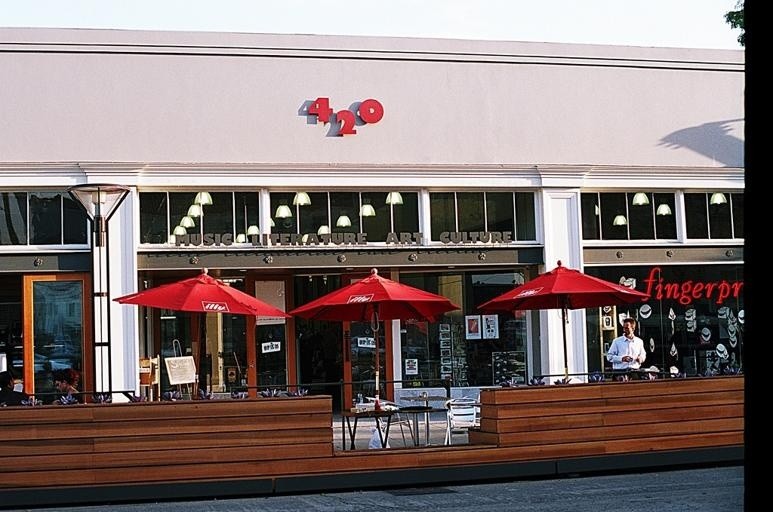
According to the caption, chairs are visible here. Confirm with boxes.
[442,397,482,446]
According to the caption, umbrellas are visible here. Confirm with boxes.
[285,267,463,403]
[475,259,653,384]
[109,267,293,394]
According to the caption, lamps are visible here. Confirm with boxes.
[168,192,403,243]
[612,192,727,227]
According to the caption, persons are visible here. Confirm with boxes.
[606,316,647,381]
[4,331,18,378]
[0,369,32,409]
[50,368,82,406]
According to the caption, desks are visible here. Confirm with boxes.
[341,396,454,450]
